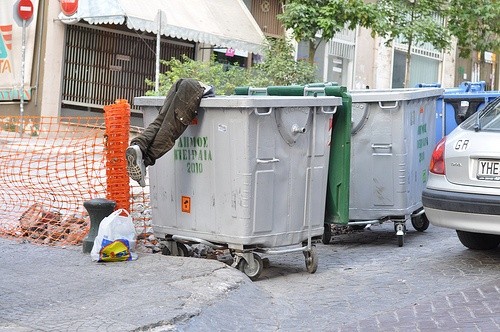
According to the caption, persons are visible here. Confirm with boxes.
[125,79,215,187]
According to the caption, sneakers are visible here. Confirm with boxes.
[124,143,146,186]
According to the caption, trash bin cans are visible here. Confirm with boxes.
[346,87,444,249]
[416,80,500,145]
[133,82,352,281]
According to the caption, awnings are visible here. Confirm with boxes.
[57,0,271,58]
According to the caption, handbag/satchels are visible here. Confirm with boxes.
[90,209,139,262]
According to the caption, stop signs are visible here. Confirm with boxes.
[17,0,33,20]
[60,0,78,16]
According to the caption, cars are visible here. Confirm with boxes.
[422,95,500,253]
